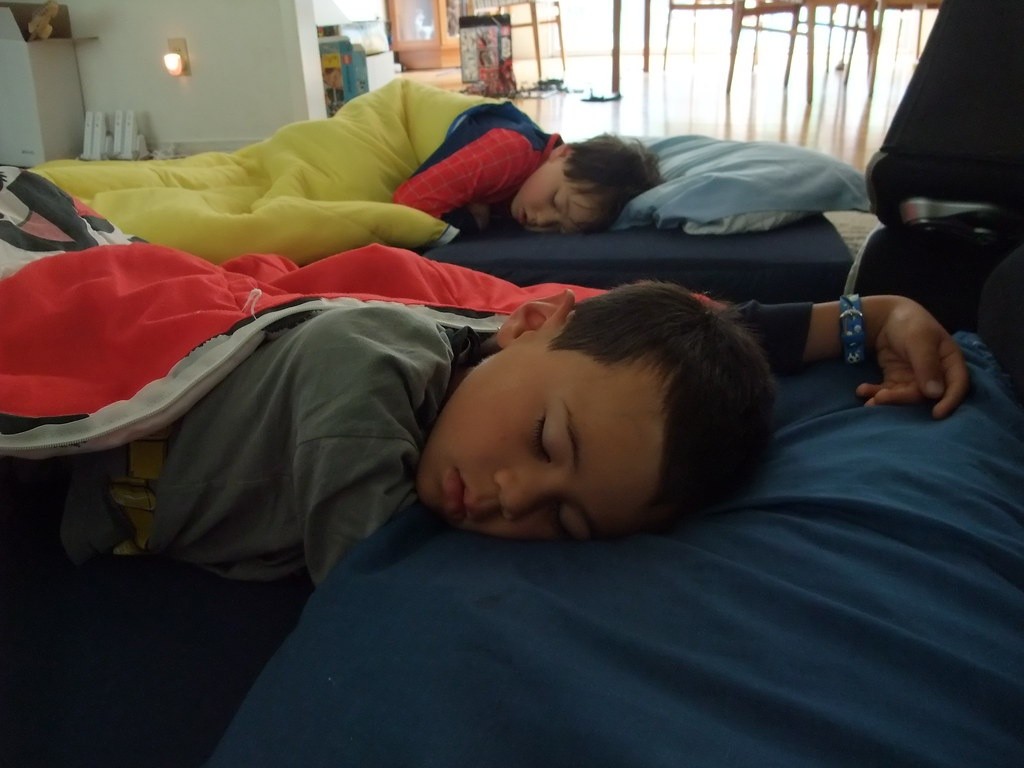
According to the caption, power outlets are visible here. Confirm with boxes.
[168,38,191,76]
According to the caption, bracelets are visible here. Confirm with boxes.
[840,290,868,364]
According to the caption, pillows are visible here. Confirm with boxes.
[610,135,871,235]
[224,330,1024,768]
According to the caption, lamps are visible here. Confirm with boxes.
[313,0,352,37]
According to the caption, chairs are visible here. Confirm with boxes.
[661,0,943,107]
[463,0,566,77]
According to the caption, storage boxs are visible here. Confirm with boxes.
[318,36,369,118]
[0,0,99,166]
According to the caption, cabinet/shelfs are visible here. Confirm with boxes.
[388,0,473,70]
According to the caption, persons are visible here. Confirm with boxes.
[1,279,968,591]
[393,102,663,234]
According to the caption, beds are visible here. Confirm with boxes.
[0,210,854,302]
[0,329,1023,767]
